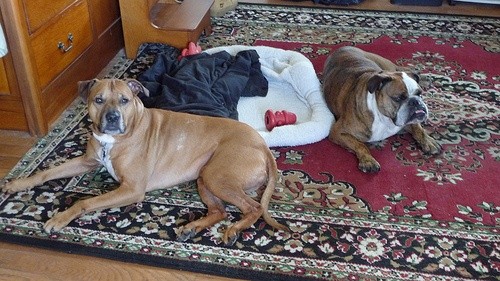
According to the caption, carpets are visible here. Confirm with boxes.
[0,4,500,281]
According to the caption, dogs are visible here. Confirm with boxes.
[0,78,294,246]
[321,46,444,174]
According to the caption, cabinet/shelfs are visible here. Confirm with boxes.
[0,0,215,138]
